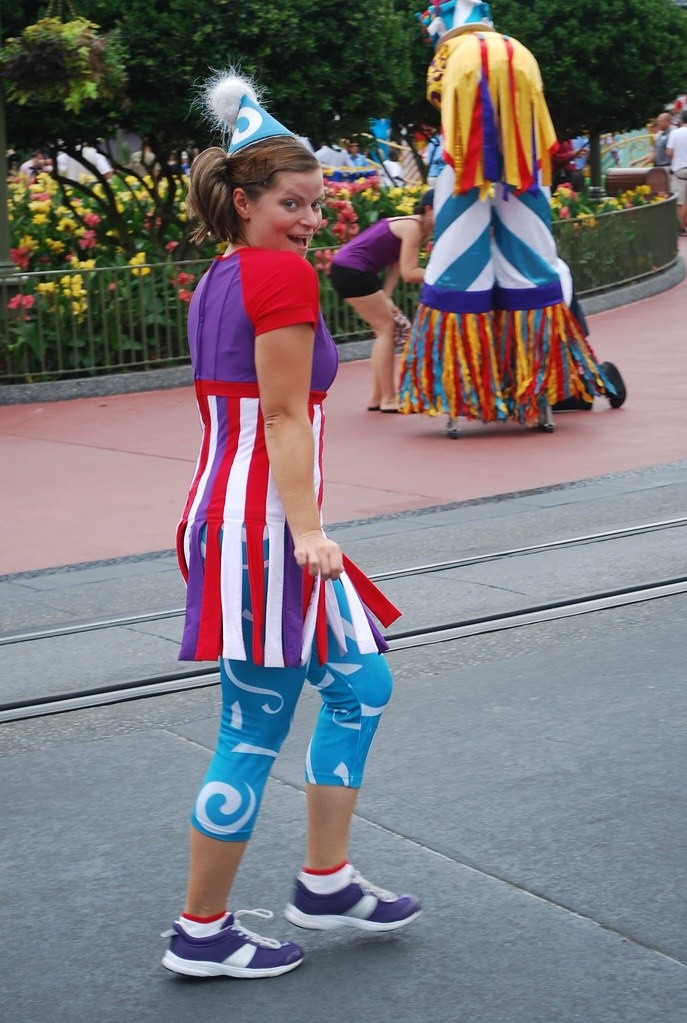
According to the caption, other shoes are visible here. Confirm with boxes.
[600,360,627,408]
[551,396,592,412]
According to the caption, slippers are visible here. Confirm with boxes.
[382,407,398,413]
[368,405,379,411]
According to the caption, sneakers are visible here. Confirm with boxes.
[283,871,424,932]
[160,909,305,978]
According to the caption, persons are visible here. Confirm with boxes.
[6,136,198,187]
[313,121,445,187]
[329,188,434,413]
[159,135,425,978]
[647,94,687,237]
[548,134,591,192]
